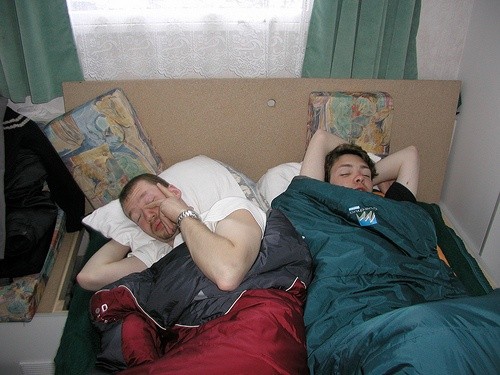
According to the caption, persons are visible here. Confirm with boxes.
[77,173,307,375]
[300,129,480,375]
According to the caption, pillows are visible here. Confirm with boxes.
[255,153,383,207]
[45,87,168,210]
[303,91,393,163]
[82,154,272,251]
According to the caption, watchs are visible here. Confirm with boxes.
[177,210,200,226]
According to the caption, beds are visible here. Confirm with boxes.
[54,80,500,375]
